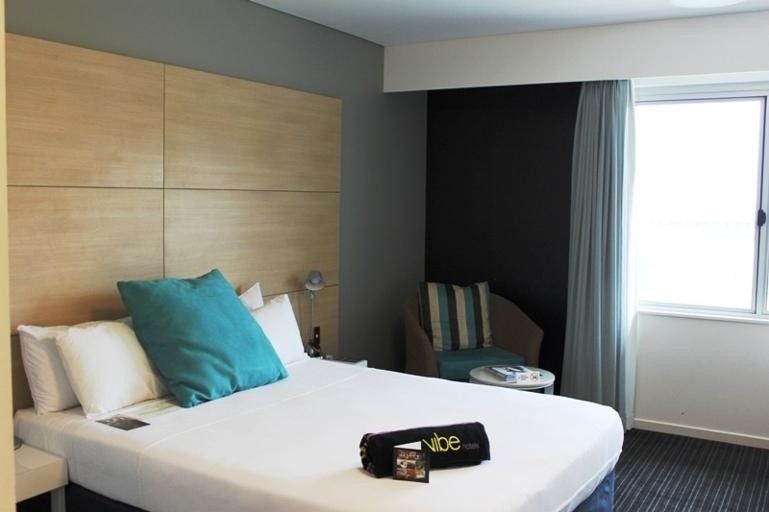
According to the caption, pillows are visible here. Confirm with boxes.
[251,293,306,365]
[238,281,263,309]
[417,280,493,352]
[116,267,288,408]
[17,316,136,417]
[54,320,173,419]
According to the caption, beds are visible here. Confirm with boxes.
[16,358,624,511]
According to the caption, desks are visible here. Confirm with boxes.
[14,443,68,512]
[469,365,555,394]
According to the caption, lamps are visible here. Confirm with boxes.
[302,268,327,358]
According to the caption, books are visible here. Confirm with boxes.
[393,440,430,484]
[485,363,545,382]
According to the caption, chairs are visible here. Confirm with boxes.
[401,287,544,384]
